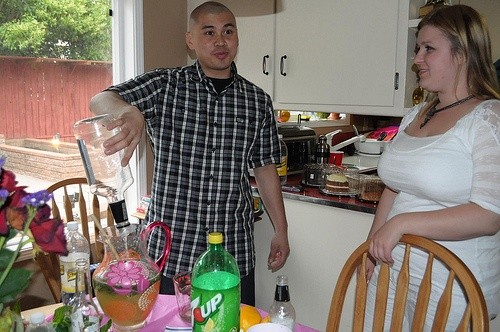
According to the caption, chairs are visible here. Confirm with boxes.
[325,234,489,332]
[35,178,119,304]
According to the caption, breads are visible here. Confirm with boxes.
[360,179,385,201]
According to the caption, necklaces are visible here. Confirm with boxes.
[419,93,475,130]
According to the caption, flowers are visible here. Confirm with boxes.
[0,154,69,332]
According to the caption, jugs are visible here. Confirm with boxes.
[92,221,171,330]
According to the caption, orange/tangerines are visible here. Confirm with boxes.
[240,304,262,332]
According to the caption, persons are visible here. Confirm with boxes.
[357,4,500,331]
[88,1,290,306]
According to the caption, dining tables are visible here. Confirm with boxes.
[20,294,320,332]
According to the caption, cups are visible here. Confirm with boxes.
[172,273,192,319]
[247,323,293,332]
[329,151,344,166]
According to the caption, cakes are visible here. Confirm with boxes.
[326,174,349,193]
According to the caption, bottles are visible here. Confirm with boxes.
[191,233,241,332]
[67,258,101,332]
[268,275,296,332]
[412,71,424,107]
[25,312,56,332]
[316,135,329,164]
[58,221,90,308]
[75,115,134,234]
[275,134,288,185]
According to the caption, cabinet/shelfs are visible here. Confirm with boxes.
[404,0,451,116]
[186,0,399,117]
